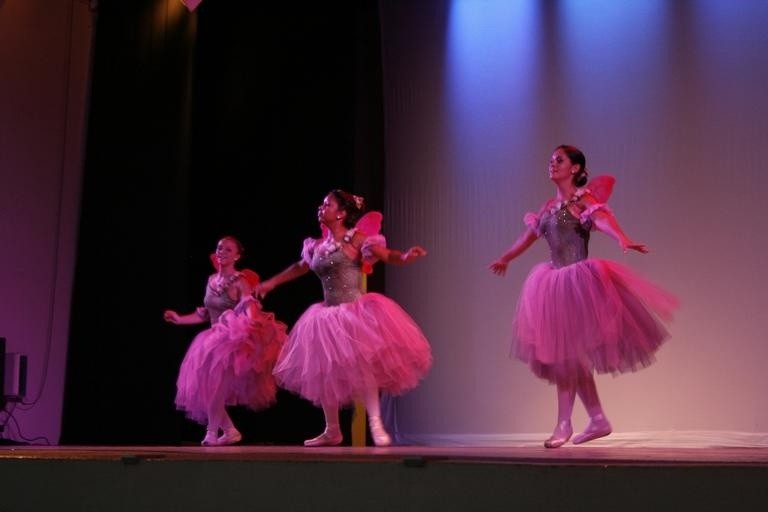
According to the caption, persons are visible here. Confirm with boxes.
[251,187,435,448]
[486,142,680,450]
[163,235,291,446]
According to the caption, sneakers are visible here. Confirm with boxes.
[200,424,220,447]
[543,419,572,448]
[217,425,242,446]
[368,416,392,447]
[572,413,612,445]
[303,423,343,448]
